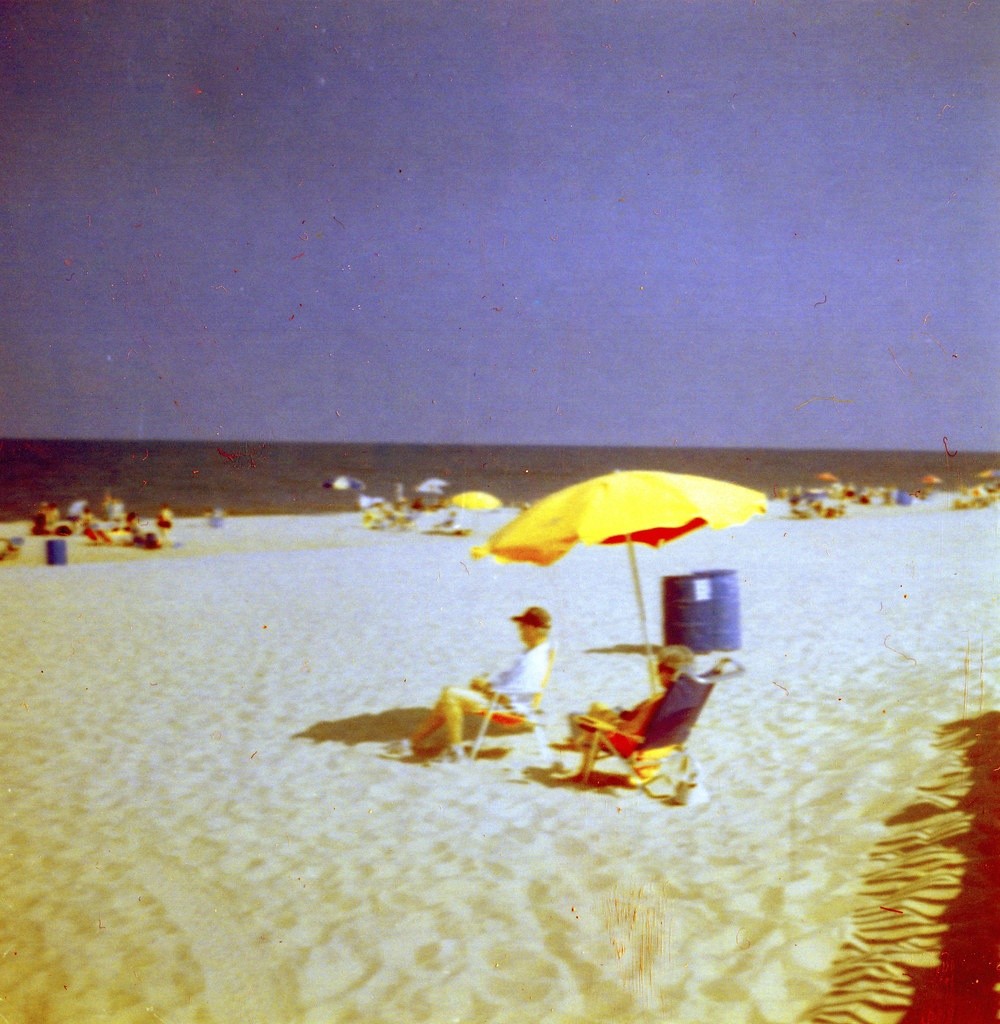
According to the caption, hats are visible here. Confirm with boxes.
[511,607,552,627]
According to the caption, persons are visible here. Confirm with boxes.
[415,607,552,767]
[30,494,174,545]
[363,496,465,532]
[569,645,695,755]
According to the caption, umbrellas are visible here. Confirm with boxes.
[974,469,1000,479]
[322,475,366,492]
[469,468,768,695]
[922,475,940,484]
[816,472,837,488]
[452,491,502,512]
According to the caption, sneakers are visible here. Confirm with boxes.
[428,746,468,767]
[381,740,415,758]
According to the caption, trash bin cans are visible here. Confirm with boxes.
[47,539,66,566]
[896,490,911,506]
[692,568,745,654]
[660,573,718,656]
[210,506,224,527]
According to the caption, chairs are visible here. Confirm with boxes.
[573,668,717,798]
[467,650,554,759]
